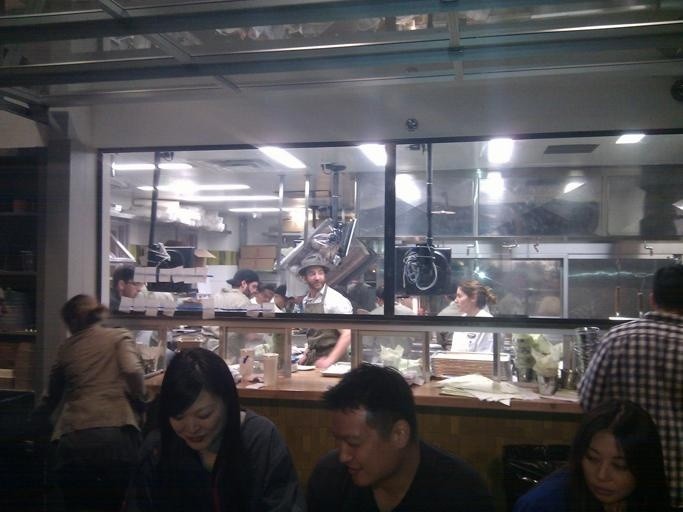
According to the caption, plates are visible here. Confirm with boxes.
[0,340,32,391]
[0,288,35,333]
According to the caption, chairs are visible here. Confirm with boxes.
[501,440,571,509]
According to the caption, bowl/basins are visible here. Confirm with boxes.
[513,326,600,397]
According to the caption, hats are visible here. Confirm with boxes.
[298,255,329,274]
[227,269,257,285]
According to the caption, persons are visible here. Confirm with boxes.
[29,294,153,512]
[512,399,670,511]
[139,346,298,511]
[305,362,490,512]
[494,268,636,319]
[578,266,682,512]
[112,255,493,372]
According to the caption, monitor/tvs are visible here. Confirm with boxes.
[395,247,451,295]
[147,248,194,292]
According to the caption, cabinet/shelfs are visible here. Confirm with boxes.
[0,148,48,392]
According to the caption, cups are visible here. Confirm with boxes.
[238,347,278,388]
